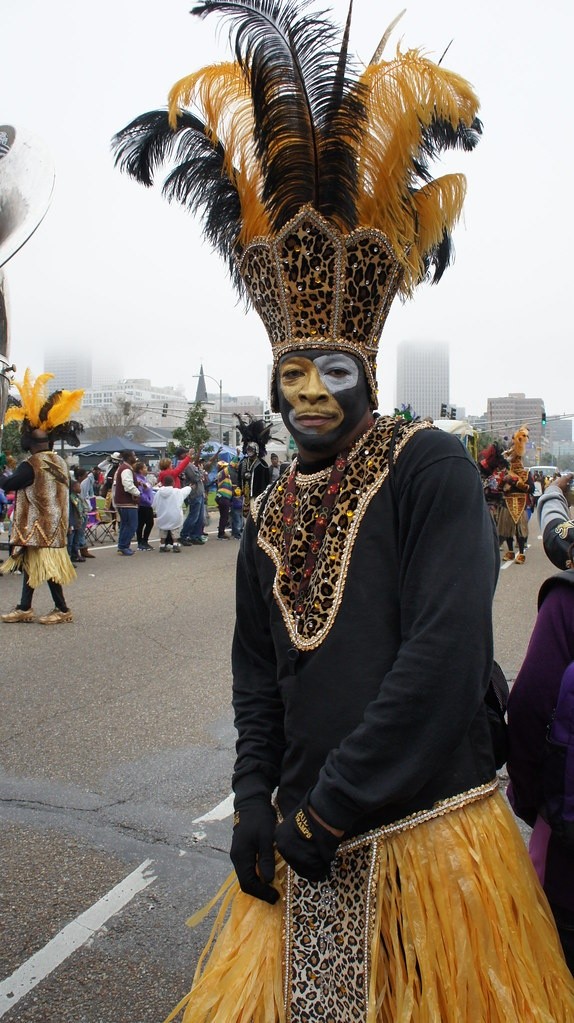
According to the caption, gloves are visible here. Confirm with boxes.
[276,786,341,881]
[230,807,280,904]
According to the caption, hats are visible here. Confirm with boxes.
[173,447,189,455]
[217,460,228,470]
[195,458,206,466]
[110,452,124,460]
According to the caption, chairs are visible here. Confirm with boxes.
[82,511,118,547]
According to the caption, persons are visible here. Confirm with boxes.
[477,455,561,564]
[537,473,574,571]
[68,442,297,565]
[505,564,574,979]
[0,428,77,624]
[180,349,574,1023]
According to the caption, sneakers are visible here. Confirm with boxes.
[1,606,37,622]
[39,607,72,625]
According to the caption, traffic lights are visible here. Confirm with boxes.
[450,407,456,420]
[541,413,546,425]
[440,403,447,417]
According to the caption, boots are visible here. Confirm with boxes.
[80,543,96,558]
[72,545,86,562]
[72,563,78,568]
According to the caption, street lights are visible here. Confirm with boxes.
[192,375,222,446]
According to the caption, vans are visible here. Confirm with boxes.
[530,466,558,476]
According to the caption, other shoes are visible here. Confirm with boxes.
[158,527,244,552]
[117,548,136,556]
[516,554,525,563]
[503,551,515,560]
[523,542,527,548]
[515,541,520,548]
[144,541,155,549]
[137,544,151,551]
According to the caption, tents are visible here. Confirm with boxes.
[71,436,159,456]
[200,442,249,492]
